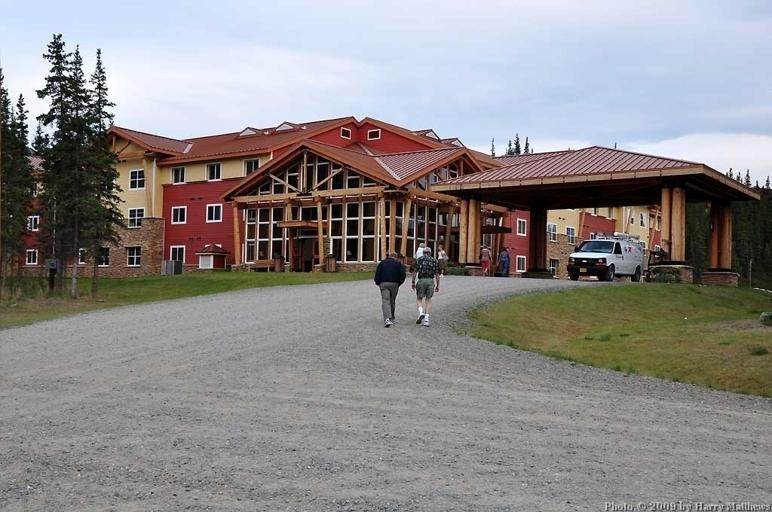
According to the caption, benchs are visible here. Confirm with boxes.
[247,260,275,272]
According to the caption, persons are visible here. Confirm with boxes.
[374,251,406,327]
[481,246,493,276]
[438,244,446,277]
[411,247,440,326]
[499,249,509,277]
[416,243,425,258]
[506,247,511,277]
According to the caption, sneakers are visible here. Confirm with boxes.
[420,319,429,326]
[383,317,396,327]
[416,312,425,323]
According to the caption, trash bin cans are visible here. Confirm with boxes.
[274,255,285,272]
[326,254,336,272]
[161,260,182,275]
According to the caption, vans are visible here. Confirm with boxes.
[566,238,644,281]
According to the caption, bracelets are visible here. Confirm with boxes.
[412,280,415,284]
